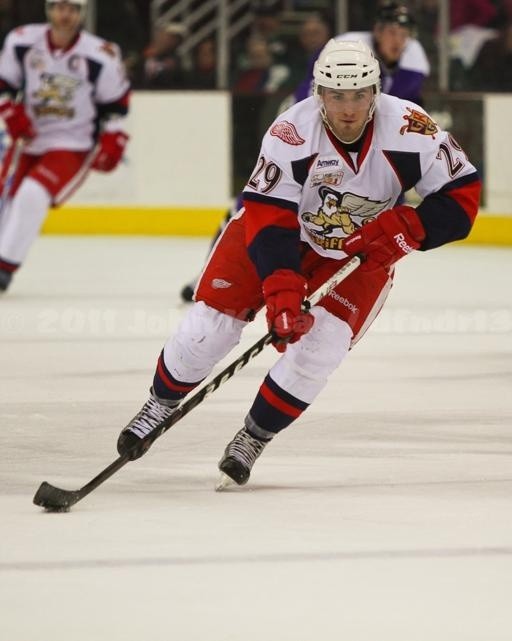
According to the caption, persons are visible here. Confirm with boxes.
[3,2,135,301]
[181,0,434,303]
[119,1,430,175]
[112,36,483,484]
[448,0,512,93]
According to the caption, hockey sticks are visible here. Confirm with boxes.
[34,252,364,512]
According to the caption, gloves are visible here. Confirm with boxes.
[0,95,39,141]
[89,120,132,173]
[259,269,316,353]
[338,206,428,274]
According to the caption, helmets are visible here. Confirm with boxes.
[375,4,419,37]
[311,39,382,124]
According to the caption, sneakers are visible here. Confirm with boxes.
[117,386,180,463]
[216,425,274,486]
